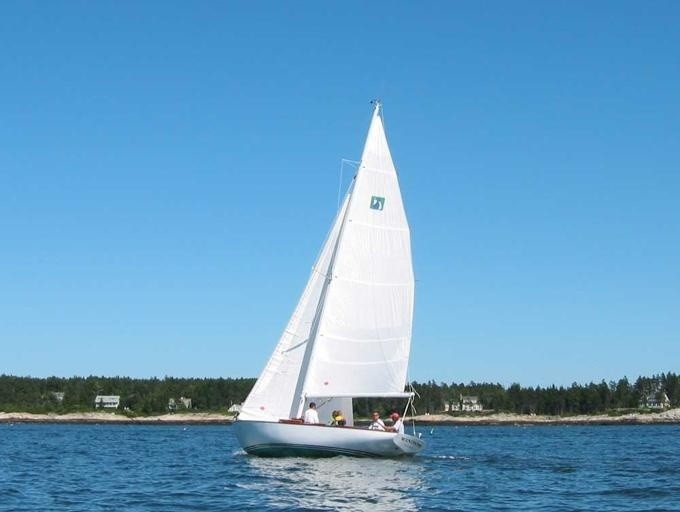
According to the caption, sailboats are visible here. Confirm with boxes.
[230,95,429,458]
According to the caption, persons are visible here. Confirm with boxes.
[327,410,346,426]
[304,402,319,424]
[368,410,385,431]
[382,413,404,434]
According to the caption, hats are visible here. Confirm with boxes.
[390,413,399,418]
